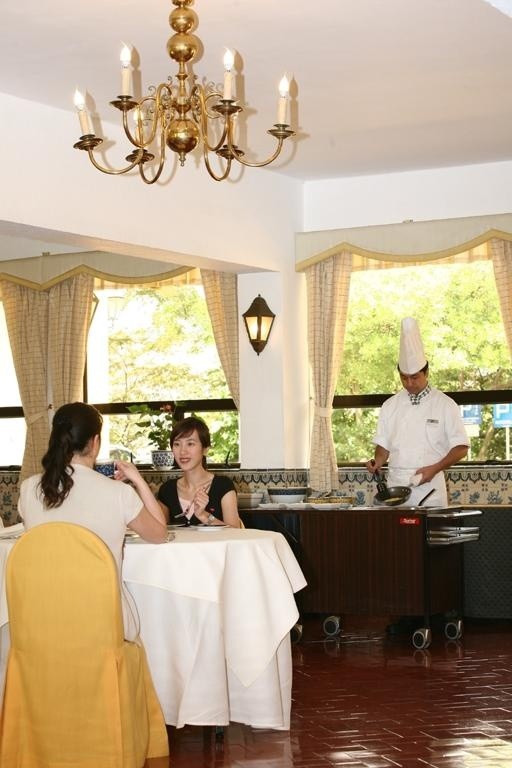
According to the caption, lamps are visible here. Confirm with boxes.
[70,0,297,183]
[243,292,276,356]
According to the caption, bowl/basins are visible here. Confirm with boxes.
[308,497,330,503]
[330,496,355,504]
[267,487,306,504]
[236,494,264,509]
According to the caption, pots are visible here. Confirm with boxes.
[372,483,414,506]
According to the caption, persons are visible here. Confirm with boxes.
[16,401,168,644]
[365,318,469,510]
[155,415,241,528]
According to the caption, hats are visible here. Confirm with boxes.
[398,316,428,375]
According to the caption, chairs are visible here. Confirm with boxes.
[0,521,169,767]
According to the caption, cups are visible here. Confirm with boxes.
[95,462,115,478]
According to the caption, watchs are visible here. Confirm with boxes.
[204,513,216,526]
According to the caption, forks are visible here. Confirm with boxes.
[174,488,203,519]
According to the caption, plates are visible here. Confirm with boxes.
[285,503,312,509]
[259,503,285,509]
[313,504,342,510]
[125,530,140,538]
[191,523,230,531]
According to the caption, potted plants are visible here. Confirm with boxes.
[126,397,189,470]
